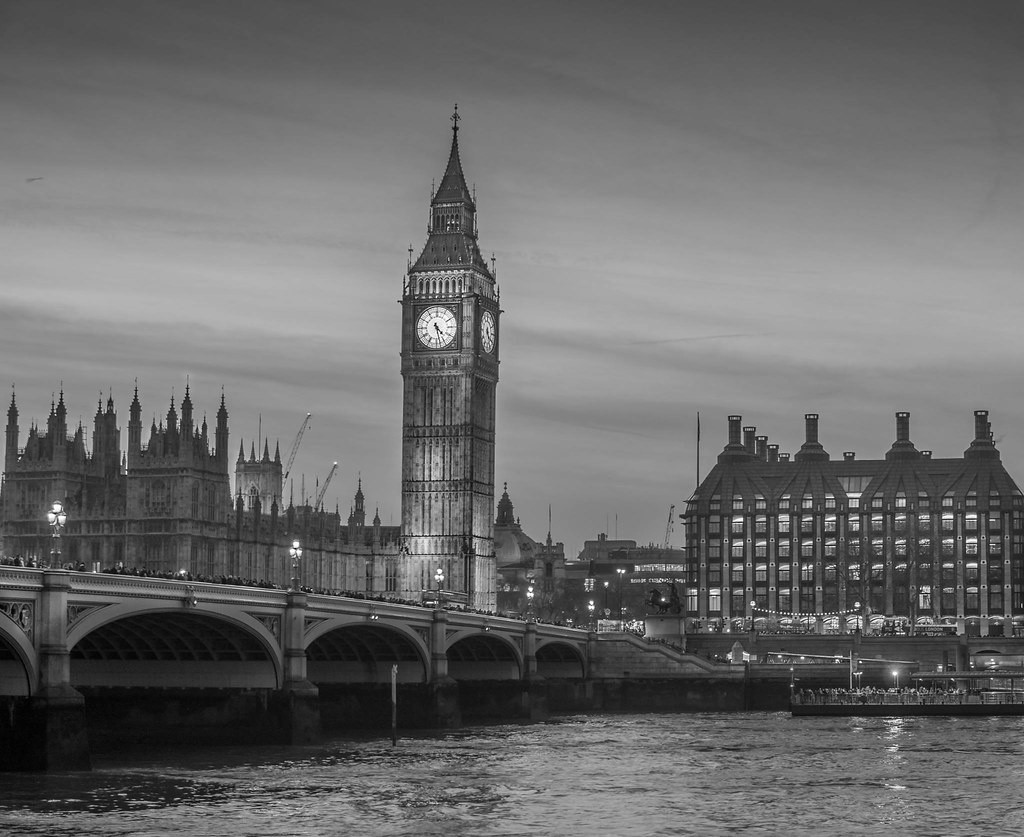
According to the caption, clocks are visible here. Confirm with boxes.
[481,310,496,354]
[415,305,457,350]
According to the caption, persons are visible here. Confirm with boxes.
[891,620,899,636]
[300,585,597,632]
[799,686,970,704]
[0,554,276,590]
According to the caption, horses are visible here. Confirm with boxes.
[644,588,672,615]
